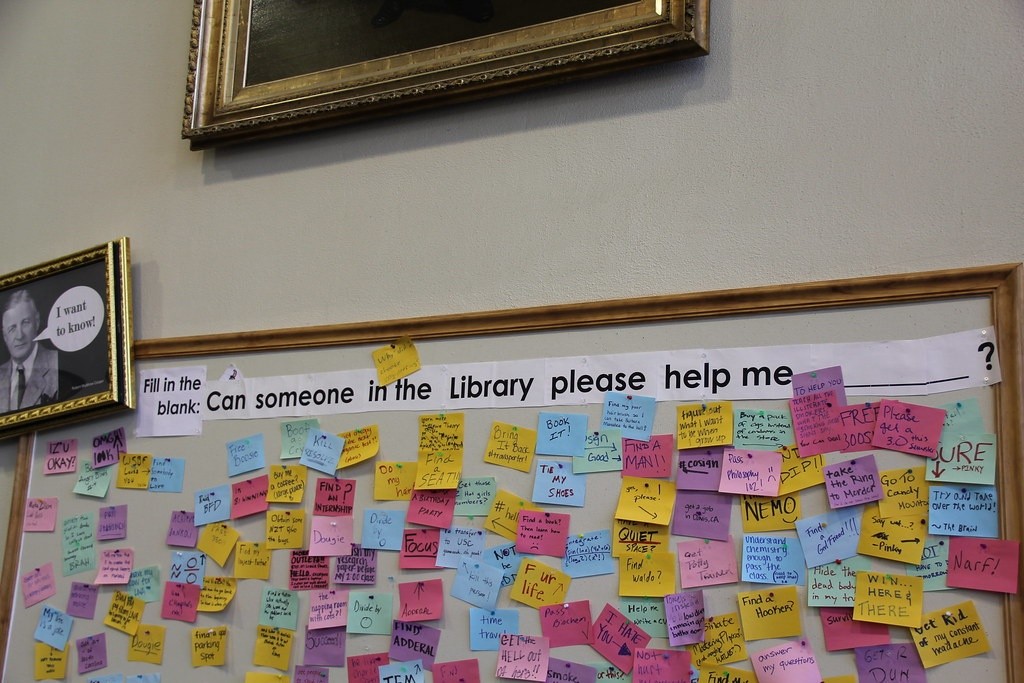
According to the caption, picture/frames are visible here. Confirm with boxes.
[0,236,133,437]
[1,263,1022,683]
[180,0,708,149]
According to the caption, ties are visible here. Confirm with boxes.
[16,365,25,410]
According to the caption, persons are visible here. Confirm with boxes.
[0,289,59,414]
